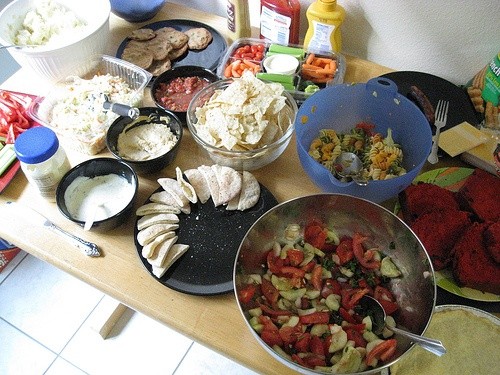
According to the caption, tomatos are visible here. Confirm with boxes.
[241,224,402,373]
[233,43,265,62]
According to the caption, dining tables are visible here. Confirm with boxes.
[1,1,500,375]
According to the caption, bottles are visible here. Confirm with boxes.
[259,0,300,47]
[303,0,348,57]
[225,0,251,43]
[15,126,73,202]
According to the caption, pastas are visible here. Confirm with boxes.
[306,126,407,182]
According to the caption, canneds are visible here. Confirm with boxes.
[225,0,251,41]
[302,0,347,53]
[257,0,300,47]
[15,126,71,203]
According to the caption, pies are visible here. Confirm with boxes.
[136,164,260,279]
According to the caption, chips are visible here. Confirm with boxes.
[192,72,294,151]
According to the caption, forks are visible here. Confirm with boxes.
[425,99,449,163]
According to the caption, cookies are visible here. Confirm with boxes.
[121,26,213,76]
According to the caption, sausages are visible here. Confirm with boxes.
[411,85,435,121]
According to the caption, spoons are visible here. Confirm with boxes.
[353,290,447,357]
[333,150,369,186]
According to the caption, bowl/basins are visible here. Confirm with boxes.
[186,76,299,170]
[106,104,184,176]
[293,76,434,206]
[55,156,139,234]
[150,65,222,126]
[110,0,169,22]
[230,187,438,373]
[0,0,112,81]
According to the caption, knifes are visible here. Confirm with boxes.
[6,201,104,259]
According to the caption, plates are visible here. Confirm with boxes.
[394,163,500,307]
[118,18,229,86]
[377,301,500,374]
[370,72,478,163]
[136,167,281,294]
[217,34,348,104]
